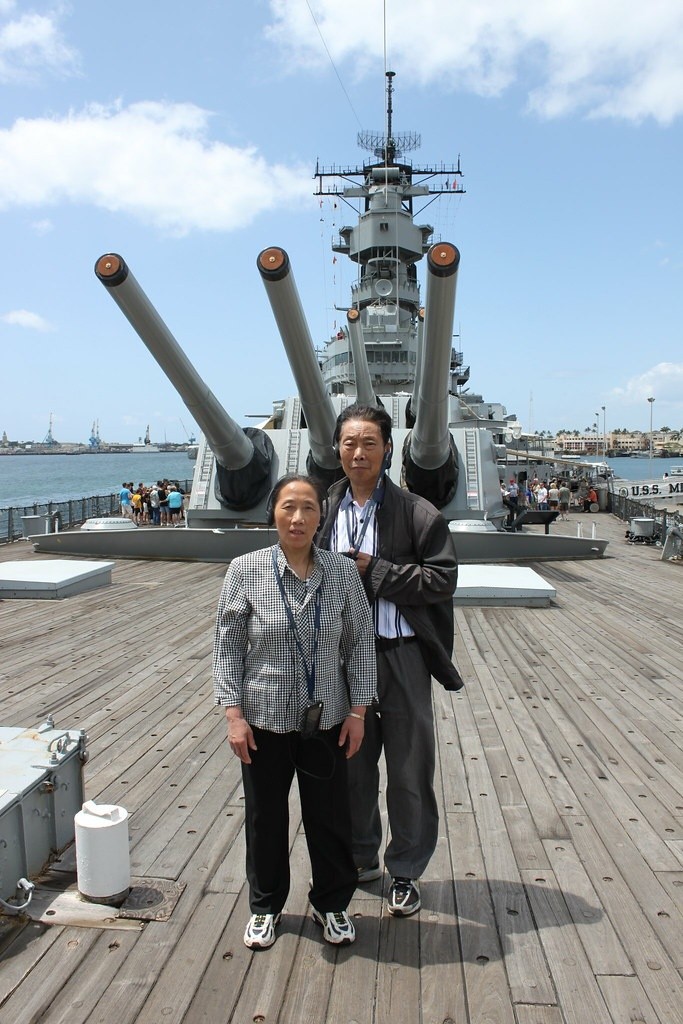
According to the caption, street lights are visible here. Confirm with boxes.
[594,412,599,462]
[601,408,606,460]
[646,397,654,459]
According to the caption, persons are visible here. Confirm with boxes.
[548,483,559,522]
[508,479,519,512]
[118,479,185,528]
[559,483,571,522]
[526,486,534,513]
[661,472,674,499]
[500,483,510,508]
[212,474,379,950]
[313,402,465,918]
[535,482,548,511]
[583,485,597,513]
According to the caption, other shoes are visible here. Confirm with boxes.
[134,520,182,528]
[181,517,186,521]
[584,509,590,514]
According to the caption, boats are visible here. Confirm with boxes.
[561,454,580,459]
[670,465,683,477]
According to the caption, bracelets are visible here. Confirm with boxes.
[347,712,364,721]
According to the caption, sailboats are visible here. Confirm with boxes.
[127,424,161,453]
[175,417,201,452]
[36,411,67,454]
[78,419,111,453]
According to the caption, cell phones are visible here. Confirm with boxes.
[303,702,325,740]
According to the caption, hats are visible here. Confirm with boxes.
[500,482,506,488]
[152,486,157,490]
[509,479,515,484]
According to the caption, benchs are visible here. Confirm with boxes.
[590,503,599,512]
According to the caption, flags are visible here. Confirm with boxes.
[446,179,449,190]
[452,178,456,190]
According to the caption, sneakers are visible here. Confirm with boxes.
[308,905,357,945]
[387,877,422,918]
[308,858,382,890]
[243,909,284,950]
[560,517,570,521]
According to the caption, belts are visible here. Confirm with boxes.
[373,636,422,651]
